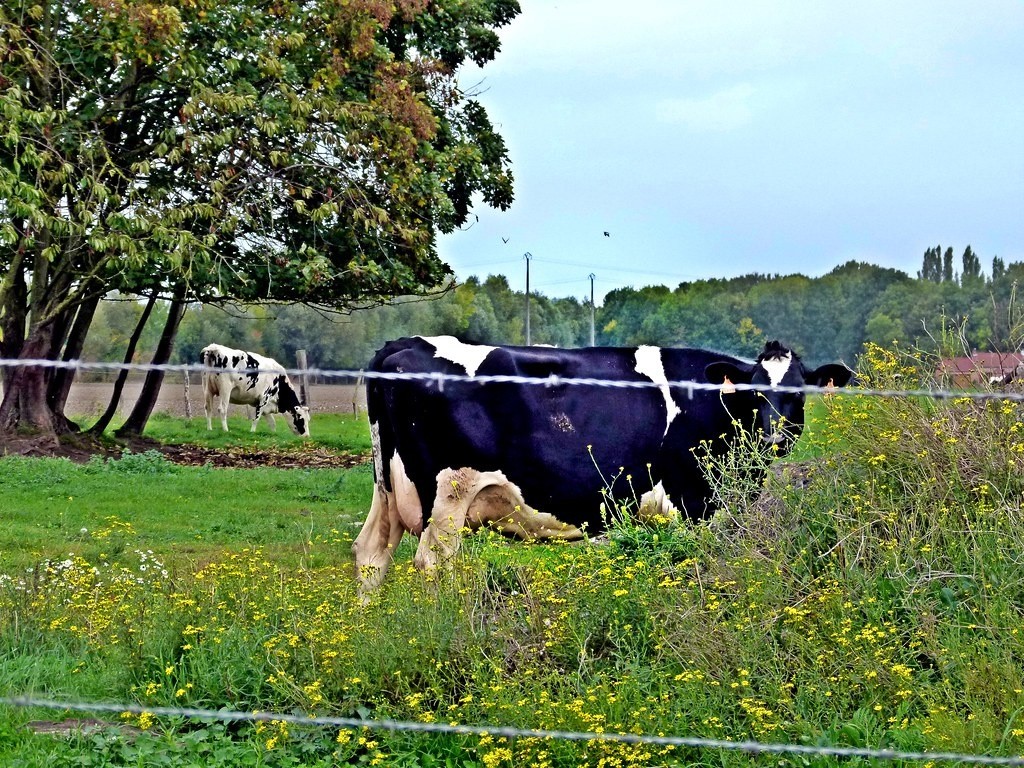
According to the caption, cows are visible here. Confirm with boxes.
[197,335,849,610]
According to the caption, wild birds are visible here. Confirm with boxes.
[603,231,610,237]
